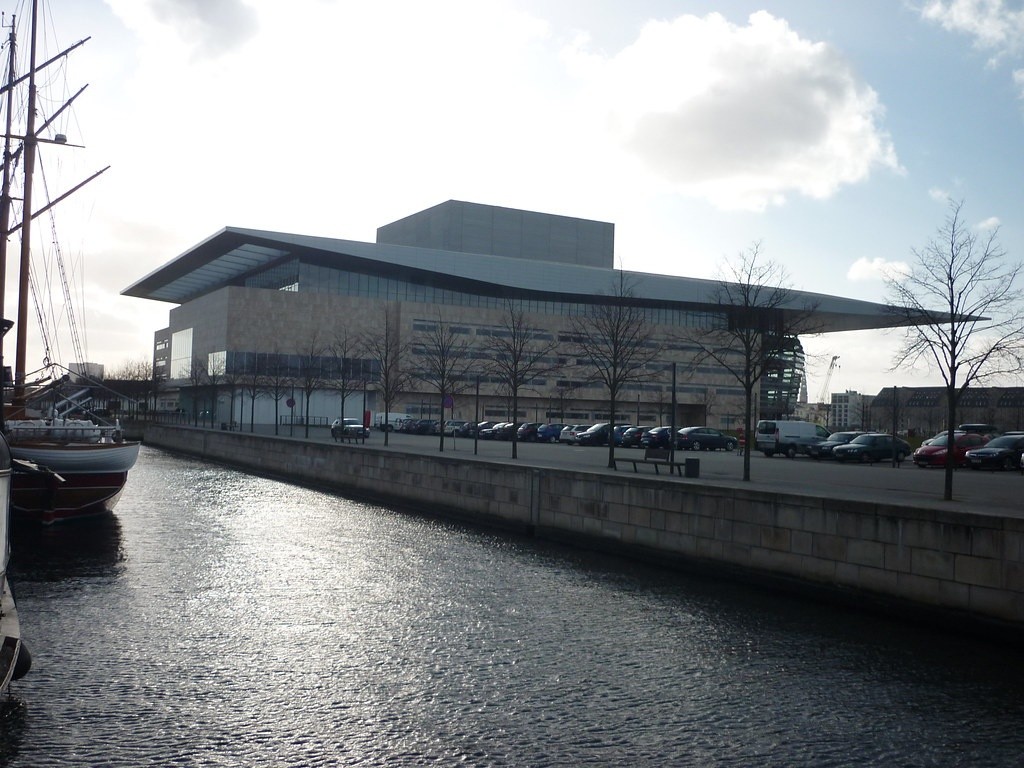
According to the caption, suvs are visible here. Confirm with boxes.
[515,422,543,441]
[559,425,592,443]
[621,426,652,449]
[537,423,567,443]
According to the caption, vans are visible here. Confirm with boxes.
[753,418,832,458]
[575,423,632,446]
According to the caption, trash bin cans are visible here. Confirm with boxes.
[220,423,227,430]
[685,458,700,478]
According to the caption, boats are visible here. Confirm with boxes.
[7,409,142,521]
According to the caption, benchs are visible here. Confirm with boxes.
[611,445,685,476]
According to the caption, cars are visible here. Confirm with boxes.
[431,421,446,434]
[674,426,737,451]
[470,421,498,437]
[607,425,631,443]
[914,424,1024,473]
[413,420,437,433]
[480,422,509,438]
[493,423,517,438]
[815,431,911,463]
[459,422,477,436]
[332,418,371,439]
[639,425,684,447]
[401,418,417,432]
[444,420,465,434]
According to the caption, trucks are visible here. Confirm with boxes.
[376,413,414,431]
